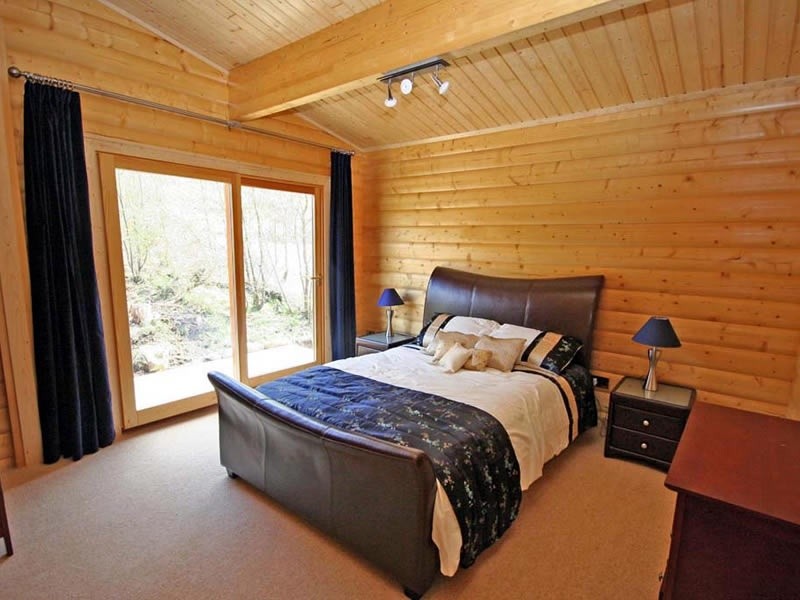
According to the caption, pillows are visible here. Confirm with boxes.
[416,312,585,371]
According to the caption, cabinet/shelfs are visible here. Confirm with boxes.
[656,400,800,600]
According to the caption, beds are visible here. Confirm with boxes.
[208,265,607,600]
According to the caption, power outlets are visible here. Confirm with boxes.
[592,374,610,390]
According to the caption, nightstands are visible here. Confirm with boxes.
[355,332,411,356]
[603,375,697,474]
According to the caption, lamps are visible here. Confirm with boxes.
[383,79,397,108]
[399,72,419,95]
[375,288,405,337]
[632,314,682,391]
[429,64,451,97]
